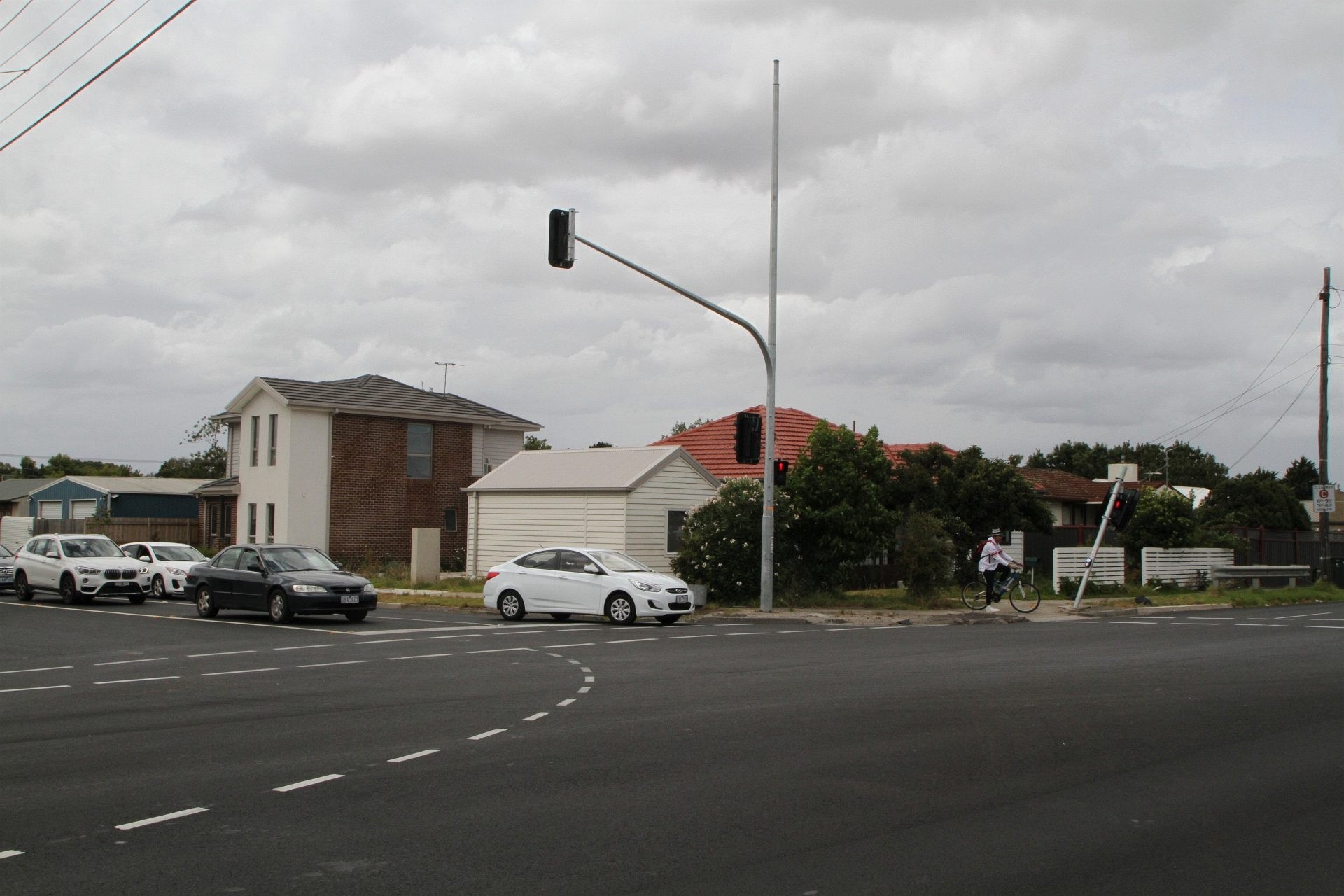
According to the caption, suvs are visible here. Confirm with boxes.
[13,533,153,607]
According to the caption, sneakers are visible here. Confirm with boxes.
[1003,586,1010,591]
[985,606,1000,612]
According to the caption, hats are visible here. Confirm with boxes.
[991,529,1003,536]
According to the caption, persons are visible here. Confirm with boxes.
[978,528,1022,612]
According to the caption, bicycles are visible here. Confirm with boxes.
[962,565,1041,614]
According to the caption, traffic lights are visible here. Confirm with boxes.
[776,459,789,486]
[732,412,762,466]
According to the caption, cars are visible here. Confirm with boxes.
[115,541,213,599]
[183,543,378,623]
[0,542,17,591]
[483,546,696,626]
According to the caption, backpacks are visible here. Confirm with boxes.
[971,539,994,563]
